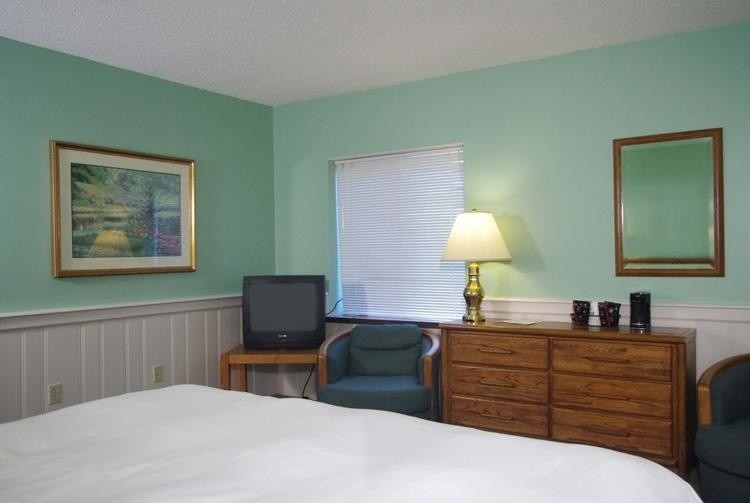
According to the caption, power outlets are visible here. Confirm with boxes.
[47,381,63,405]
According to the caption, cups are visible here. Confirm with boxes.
[629,291,650,330]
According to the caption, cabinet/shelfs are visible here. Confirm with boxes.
[219,345,318,391]
[440,318,697,480]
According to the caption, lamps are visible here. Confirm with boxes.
[439,208,514,323]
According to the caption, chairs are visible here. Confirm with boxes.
[687,349,750,503]
[319,322,442,423]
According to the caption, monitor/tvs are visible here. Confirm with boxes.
[242,275,325,350]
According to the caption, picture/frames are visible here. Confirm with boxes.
[50,136,198,279]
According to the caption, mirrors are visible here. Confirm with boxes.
[613,127,726,278]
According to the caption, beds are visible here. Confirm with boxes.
[0,382,708,503]
